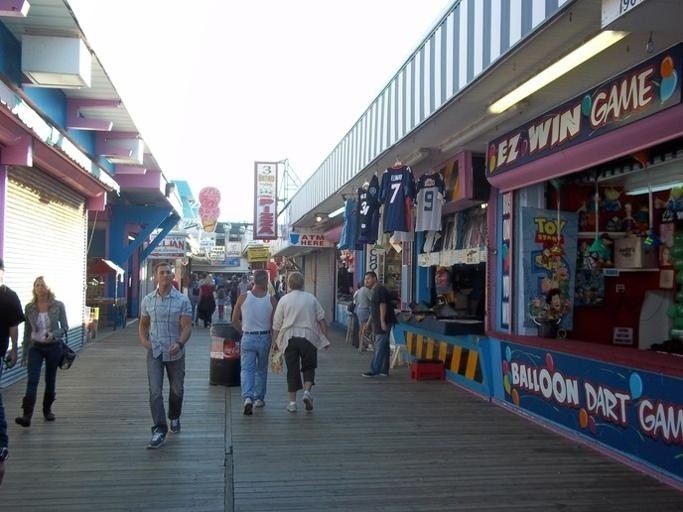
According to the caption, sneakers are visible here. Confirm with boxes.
[303,394,313,411]
[169,419,180,434]
[287,403,297,411]
[361,371,389,377]
[148,432,167,448]
[243,398,264,414]
[0,447,10,460]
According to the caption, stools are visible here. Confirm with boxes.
[411,359,445,380]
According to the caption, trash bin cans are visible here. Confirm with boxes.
[209,321,243,388]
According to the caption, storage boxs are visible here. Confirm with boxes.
[615,237,658,269]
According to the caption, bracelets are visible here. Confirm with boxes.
[175,339,185,349]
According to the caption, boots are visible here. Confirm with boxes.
[42,394,57,420]
[15,398,35,428]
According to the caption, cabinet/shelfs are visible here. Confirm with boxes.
[577,232,660,271]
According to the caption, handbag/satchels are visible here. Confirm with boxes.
[60,344,77,369]
[347,303,356,312]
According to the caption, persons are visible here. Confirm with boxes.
[230,268,276,416]
[13,275,68,425]
[357,270,397,380]
[270,272,328,415]
[0,259,26,463]
[181,271,376,352]
[136,260,194,451]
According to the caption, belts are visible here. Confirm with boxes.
[246,330,270,335]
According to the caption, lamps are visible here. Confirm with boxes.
[315,213,328,221]
[646,30,655,53]
[489,29,631,114]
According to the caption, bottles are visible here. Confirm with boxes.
[2,363,12,370]
[2,351,10,363]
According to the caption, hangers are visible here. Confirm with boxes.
[394,155,403,167]
[362,175,369,188]
